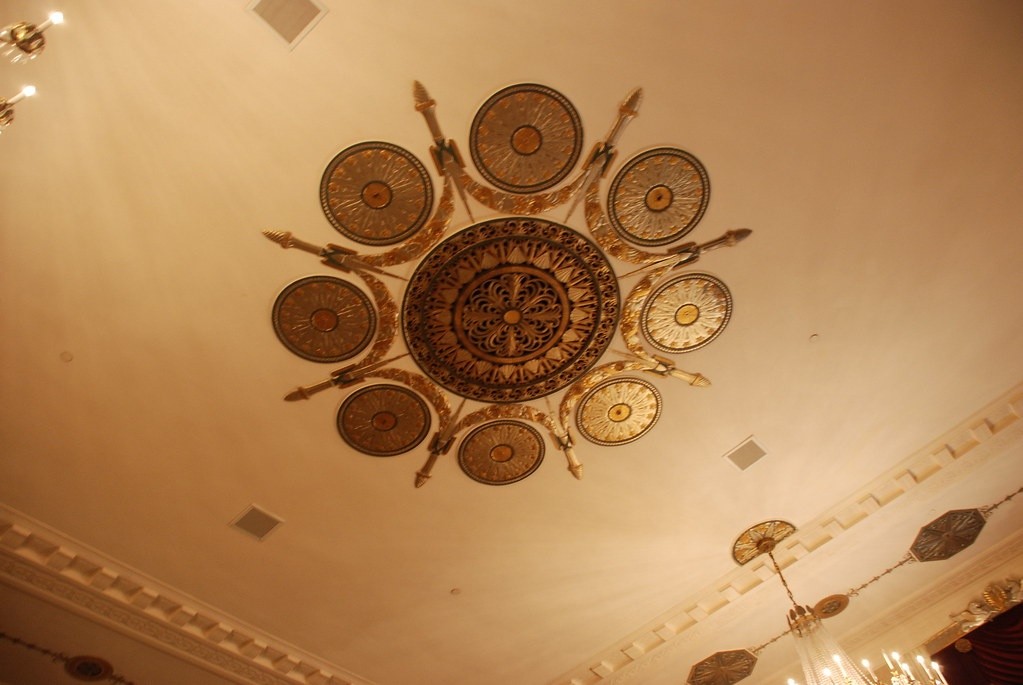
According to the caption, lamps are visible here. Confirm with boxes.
[0,9,68,128]
[733,521,948,685]
